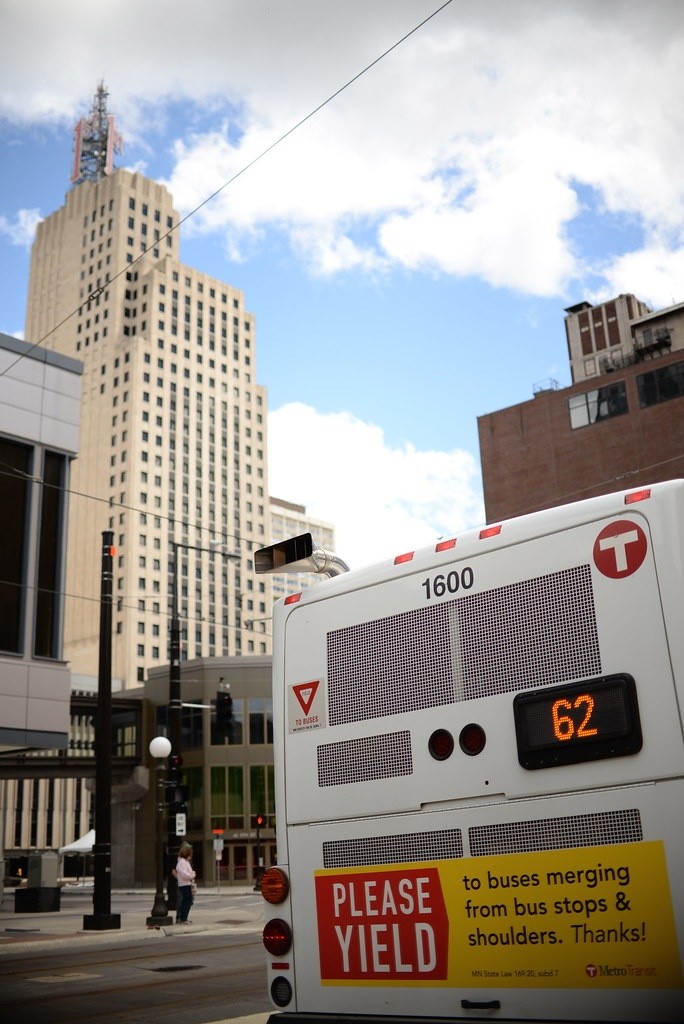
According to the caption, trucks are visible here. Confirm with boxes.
[250,479,682,1024]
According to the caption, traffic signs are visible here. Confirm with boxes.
[173,812,187,837]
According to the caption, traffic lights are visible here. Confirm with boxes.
[168,755,184,783]
[167,785,189,807]
[215,690,235,739]
[256,814,263,829]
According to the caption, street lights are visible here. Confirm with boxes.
[145,735,173,928]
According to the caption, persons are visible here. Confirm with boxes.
[171,841,197,925]
